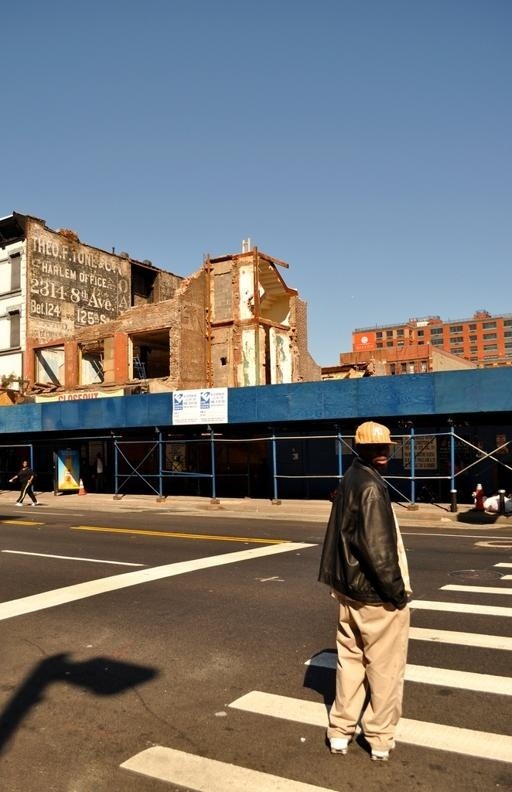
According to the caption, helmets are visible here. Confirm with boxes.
[353,419,396,446]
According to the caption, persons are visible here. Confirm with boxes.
[92,451,106,493]
[9,459,39,506]
[318,422,413,764]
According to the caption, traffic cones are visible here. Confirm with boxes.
[469,482,487,513]
[77,476,87,496]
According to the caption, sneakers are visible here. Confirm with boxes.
[31,501,39,508]
[370,739,396,762]
[327,737,350,756]
[15,501,25,509]
